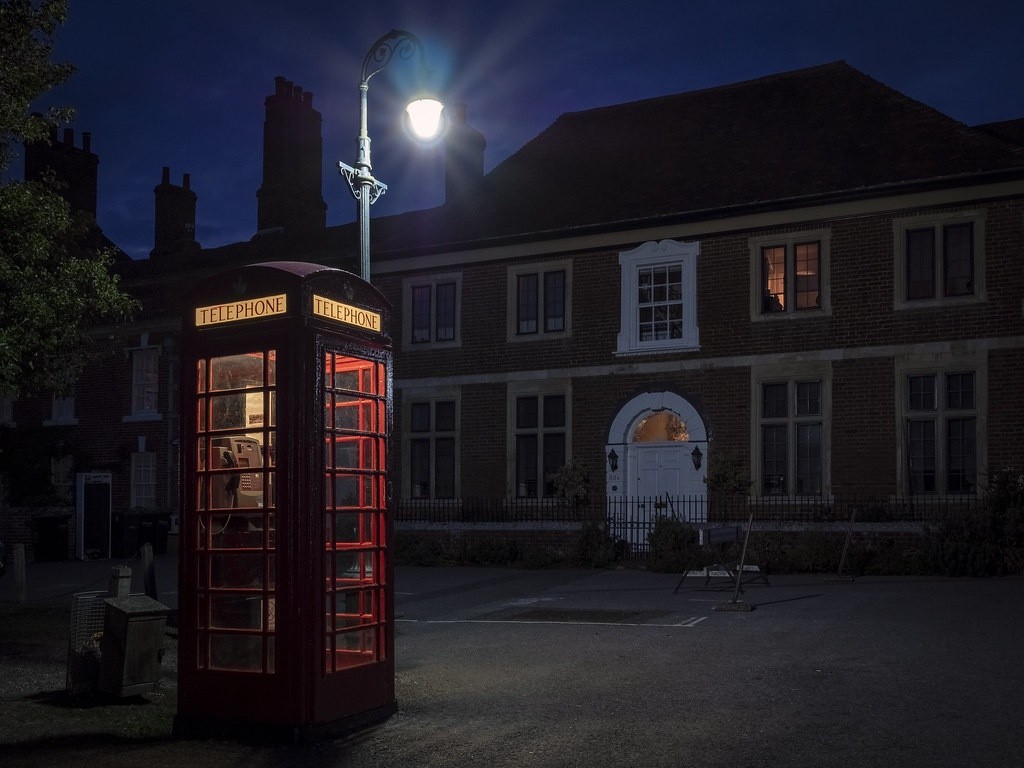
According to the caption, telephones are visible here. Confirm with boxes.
[209,435,273,518]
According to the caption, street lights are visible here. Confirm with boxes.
[335,28,459,281]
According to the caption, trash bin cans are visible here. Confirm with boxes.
[66,591,110,702]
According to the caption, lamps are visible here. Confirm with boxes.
[690,444,703,471]
[607,447,619,472]
